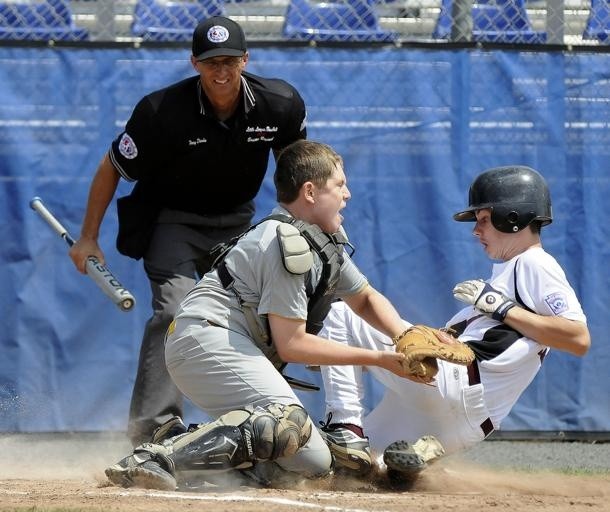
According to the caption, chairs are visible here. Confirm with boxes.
[0,0,90,41]
[433,0,547,43]
[282,0,400,42]
[130,0,224,42]
[583,0,610,47]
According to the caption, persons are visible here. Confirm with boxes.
[69,16,307,452]
[105,139,476,491]
[309,165,592,489]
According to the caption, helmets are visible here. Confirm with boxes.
[451,163,555,234]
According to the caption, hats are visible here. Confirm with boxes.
[191,14,248,63]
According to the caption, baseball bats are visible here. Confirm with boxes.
[29,197,136,312]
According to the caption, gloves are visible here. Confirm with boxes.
[451,277,520,323]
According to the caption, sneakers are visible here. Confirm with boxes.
[378,432,446,492]
[147,414,190,445]
[104,449,179,491]
[313,425,373,479]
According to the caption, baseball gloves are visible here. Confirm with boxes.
[376,324,476,386]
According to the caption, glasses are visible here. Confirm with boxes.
[200,58,244,69]
